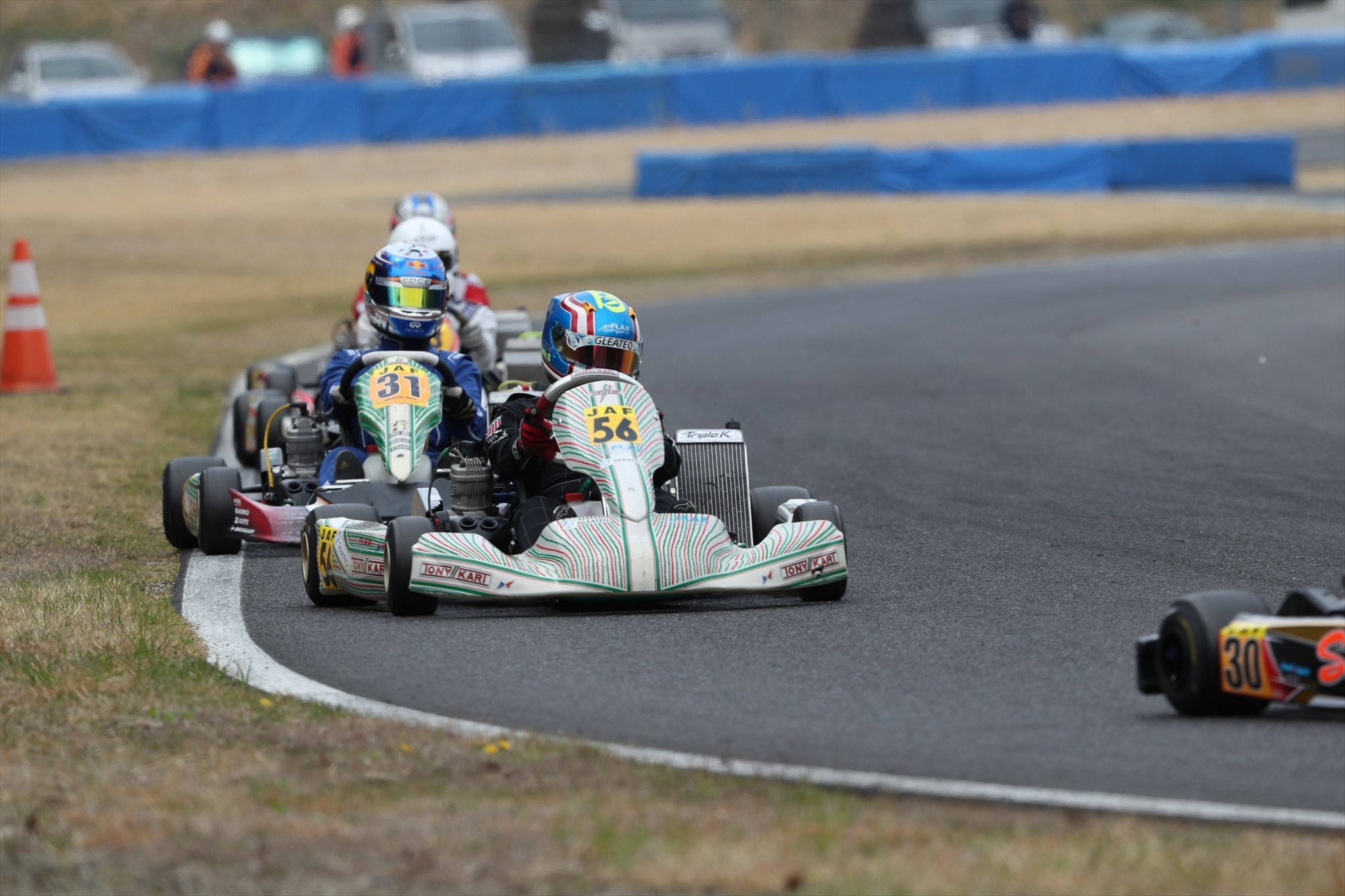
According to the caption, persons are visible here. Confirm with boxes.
[483,289,739,555]
[319,189,497,486]
[329,4,368,78]
[181,16,237,83]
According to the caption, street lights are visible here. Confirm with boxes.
[0,239,66,393]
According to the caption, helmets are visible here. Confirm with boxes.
[363,243,450,339]
[205,18,233,44]
[390,190,457,239]
[388,216,460,298]
[336,6,364,30]
[542,290,644,385]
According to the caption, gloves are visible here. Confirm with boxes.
[656,408,665,433]
[330,385,358,421]
[520,404,552,449]
[440,379,477,421]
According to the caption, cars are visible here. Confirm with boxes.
[355,3,530,76]
[8,40,151,94]
[528,0,747,65]
[854,1,1070,48]
